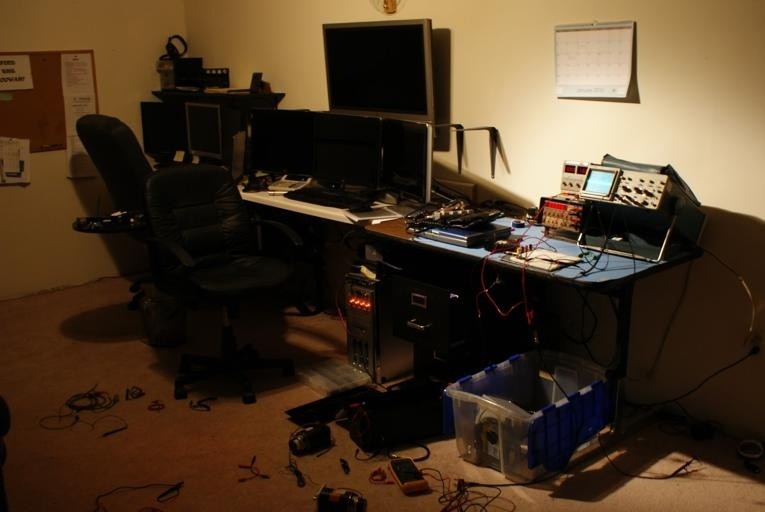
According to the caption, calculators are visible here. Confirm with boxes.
[268,174,312,191]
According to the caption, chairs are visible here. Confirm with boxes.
[72,115,234,347]
[146,163,308,404]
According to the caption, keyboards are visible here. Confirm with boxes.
[283,186,360,208]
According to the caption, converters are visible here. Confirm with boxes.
[388,456,430,494]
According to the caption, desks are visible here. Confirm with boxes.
[233,168,684,387]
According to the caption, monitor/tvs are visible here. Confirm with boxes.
[322,18,439,141]
[245,107,311,178]
[308,108,382,190]
[383,111,433,205]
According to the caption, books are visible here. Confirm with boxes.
[343,206,403,223]
[203,87,250,95]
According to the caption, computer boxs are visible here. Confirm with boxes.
[344,266,416,384]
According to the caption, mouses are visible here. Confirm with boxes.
[348,200,372,213]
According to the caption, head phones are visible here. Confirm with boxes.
[165,33,189,61]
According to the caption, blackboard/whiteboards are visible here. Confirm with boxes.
[0,49,99,153]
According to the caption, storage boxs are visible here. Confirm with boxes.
[438,344,614,484]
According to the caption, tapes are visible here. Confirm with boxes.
[512,220,525,228]
